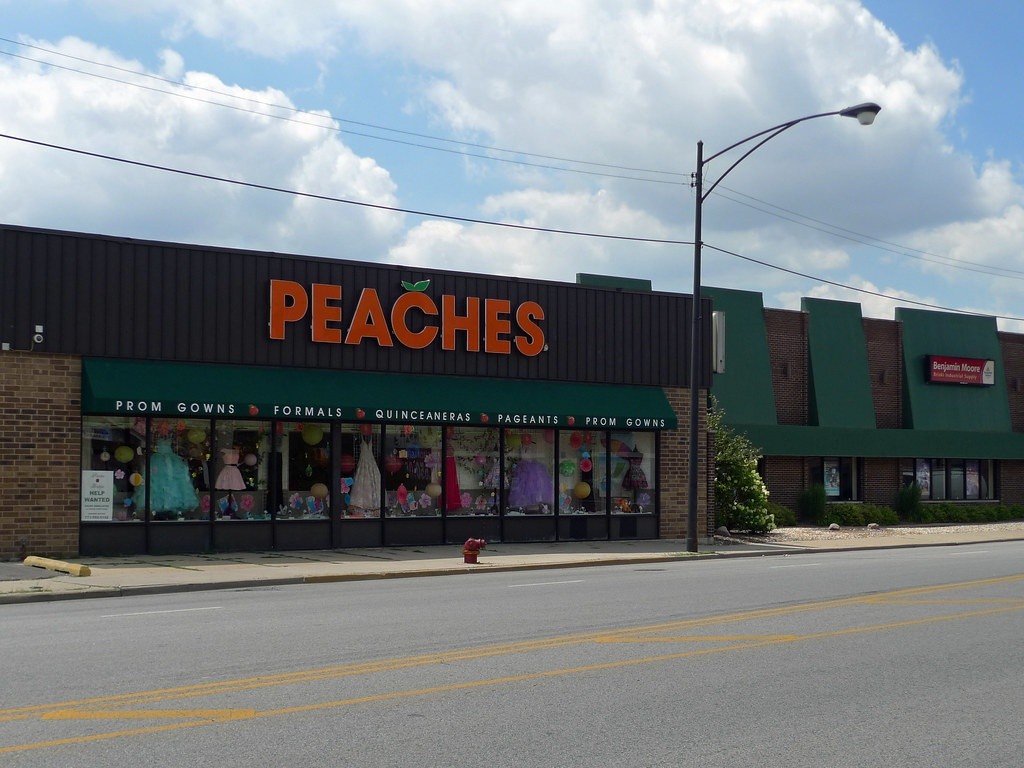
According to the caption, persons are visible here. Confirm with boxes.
[439,436,461,512]
[350,433,390,515]
[580,444,596,513]
[132,430,200,515]
[508,441,553,514]
[623,445,648,505]
[215,448,246,490]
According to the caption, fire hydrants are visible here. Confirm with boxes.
[462,538,487,564]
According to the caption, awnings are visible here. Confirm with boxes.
[82,358,678,430]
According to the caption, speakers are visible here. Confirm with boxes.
[712,310,725,374]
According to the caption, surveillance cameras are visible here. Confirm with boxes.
[34,334,43,344]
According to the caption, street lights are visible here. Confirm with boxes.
[685,102,882,552]
